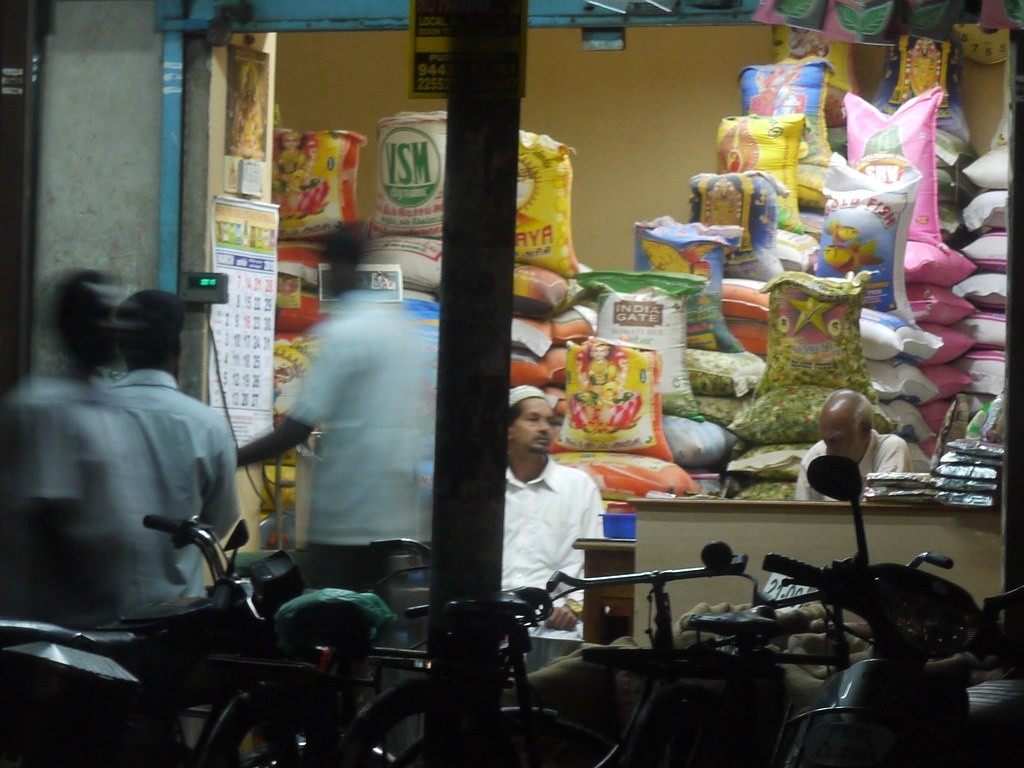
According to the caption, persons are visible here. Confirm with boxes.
[236,224,438,678]
[6,269,235,621]
[500,383,605,670]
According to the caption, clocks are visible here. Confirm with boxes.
[955,24,1010,64]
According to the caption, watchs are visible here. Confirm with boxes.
[566,600,583,621]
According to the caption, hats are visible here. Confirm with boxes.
[509,385,551,408]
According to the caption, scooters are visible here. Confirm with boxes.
[759,455,1023,768]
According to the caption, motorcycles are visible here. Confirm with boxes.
[0,509,306,768]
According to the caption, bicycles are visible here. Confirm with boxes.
[332,540,953,767]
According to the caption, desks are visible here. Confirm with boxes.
[572,540,635,643]
[629,498,1002,638]
